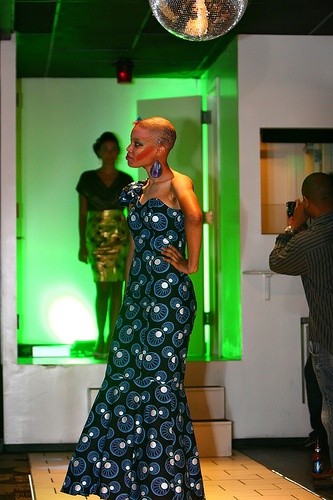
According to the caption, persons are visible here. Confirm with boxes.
[60,116,206,500]
[268,173,333,464]
[75,130,134,360]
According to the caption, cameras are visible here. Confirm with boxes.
[286,201,297,217]
